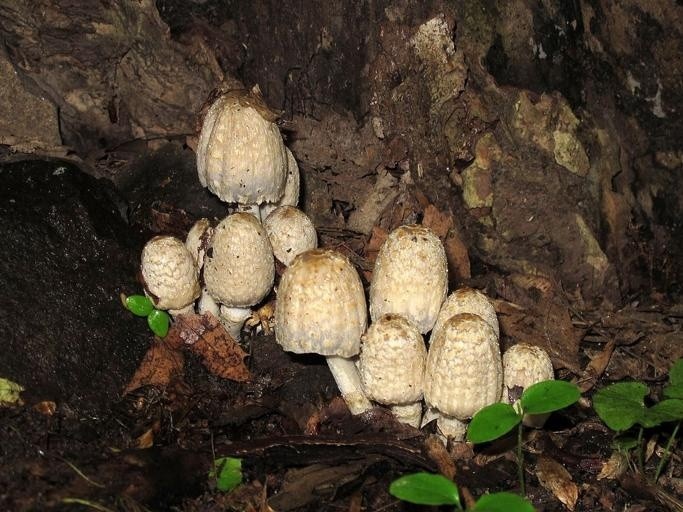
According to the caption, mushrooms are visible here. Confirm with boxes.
[138,89,555,451]
